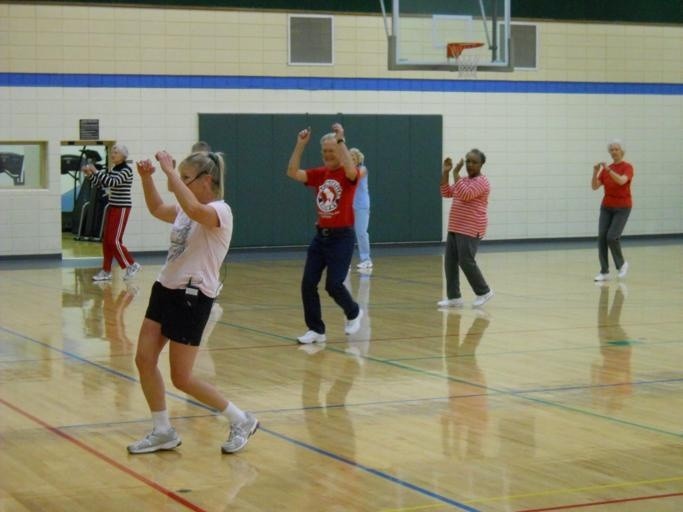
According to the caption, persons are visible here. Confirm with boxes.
[125,149,261,454]
[437,148,495,307]
[438,305,497,465]
[180,342,220,448]
[91,279,144,412]
[344,267,377,361]
[80,141,144,282]
[207,453,263,512]
[350,146,374,268]
[591,138,634,282]
[191,141,212,152]
[295,343,365,510]
[591,279,632,414]
[283,122,367,346]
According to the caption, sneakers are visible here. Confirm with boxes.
[127,426,182,453]
[123,262,141,279]
[93,270,112,281]
[595,272,610,281]
[357,260,373,269]
[297,330,326,344]
[344,309,363,335]
[438,298,462,306]
[472,290,493,306]
[617,263,628,277]
[221,412,260,454]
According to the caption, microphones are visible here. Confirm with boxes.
[186,170,208,186]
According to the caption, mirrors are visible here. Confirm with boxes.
[59,145,107,259]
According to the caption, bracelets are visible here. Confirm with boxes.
[607,168,612,172]
[337,136,346,143]
